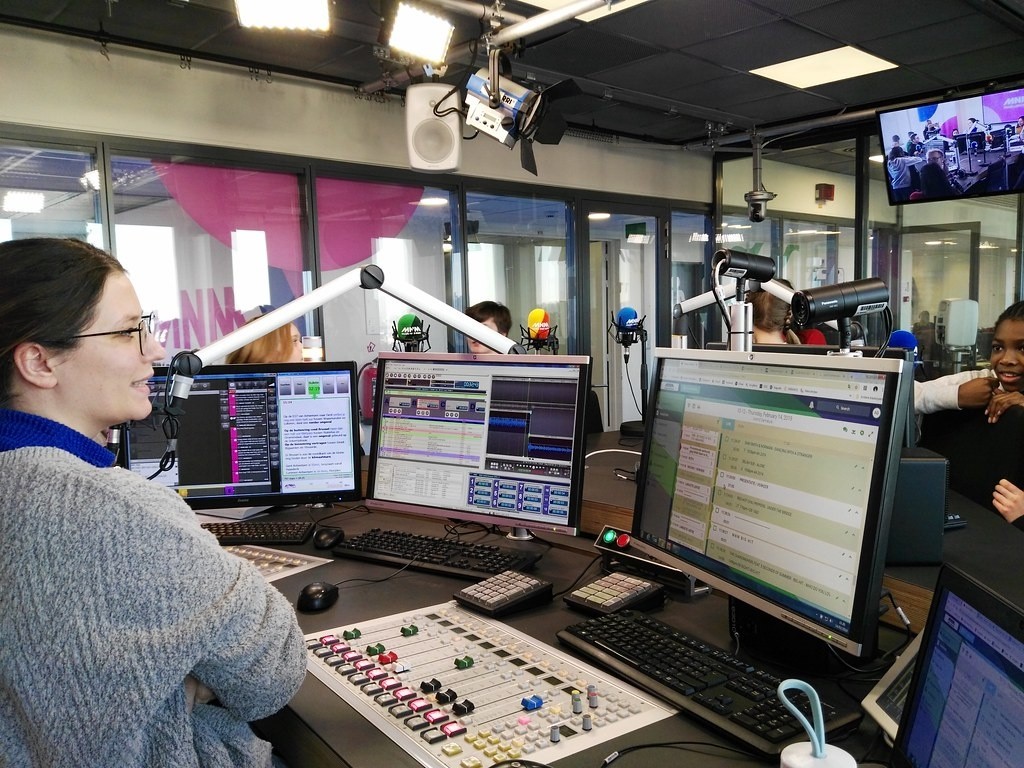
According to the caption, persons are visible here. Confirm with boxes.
[464,301,511,354]
[887,115,1024,200]
[913,299,1024,529]
[225,314,304,364]
[0,237,308,768]
[744,279,826,347]
[913,311,935,333]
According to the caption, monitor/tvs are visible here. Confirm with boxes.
[924,140,944,156]
[630,348,915,655]
[364,351,593,536]
[876,85,1024,206]
[889,563,1024,768]
[953,132,986,154]
[990,126,1015,149]
[170,361,362,521]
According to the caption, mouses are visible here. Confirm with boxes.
[313,527,346,550]
[297,582,339,613]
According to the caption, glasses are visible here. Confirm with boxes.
[928,157,941,162]
[37,312,156,356]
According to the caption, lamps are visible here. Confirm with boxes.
[234,0,337,34]
[372,0,455,73]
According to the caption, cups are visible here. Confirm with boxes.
[777,679,859,768]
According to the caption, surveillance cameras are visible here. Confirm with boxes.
[744,191,773,223]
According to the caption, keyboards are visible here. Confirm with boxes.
[201,520,316,545]
[556,607,865,763]
[332,527,543,579]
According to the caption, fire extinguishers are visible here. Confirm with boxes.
[357,357,379,424]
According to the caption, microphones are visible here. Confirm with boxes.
[616,307,637,364]
[398,315,423,352]
[890,330,919,365]
[528,309,550,355]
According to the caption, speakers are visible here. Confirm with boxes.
[884,446,951,566]
[405,82,462,174]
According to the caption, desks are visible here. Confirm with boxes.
[208,430,1024,768]
